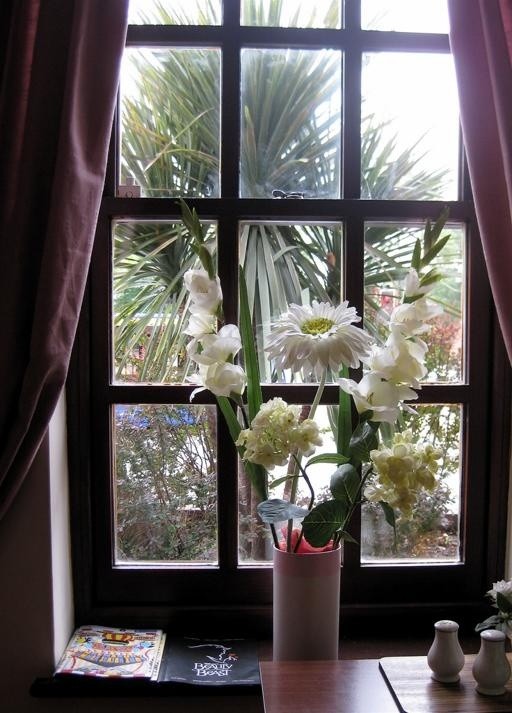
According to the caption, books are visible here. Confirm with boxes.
[157,625,261,696]
[52,625,166,697]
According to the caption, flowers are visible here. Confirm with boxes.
[468,573,512,643]
[169,192,456,553]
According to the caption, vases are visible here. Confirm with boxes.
[267,528,346,659]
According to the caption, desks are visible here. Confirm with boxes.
[255,652,511,712]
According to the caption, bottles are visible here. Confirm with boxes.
[471,628,510,697]
[426,619,464,684]
